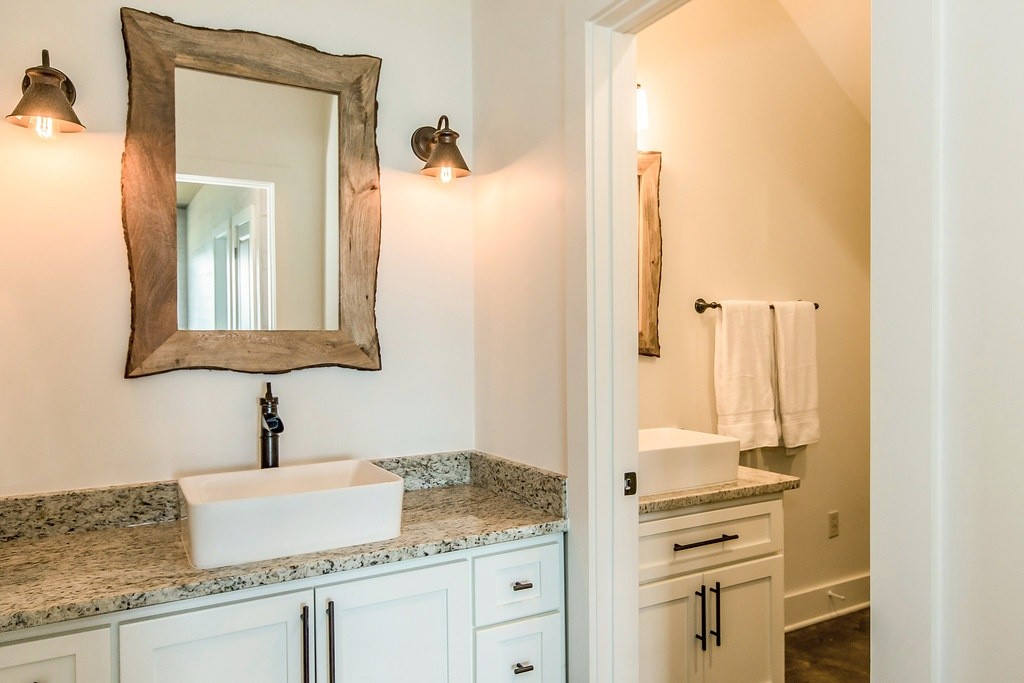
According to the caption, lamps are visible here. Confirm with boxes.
[6,49,87,143]
[412,115,471,188]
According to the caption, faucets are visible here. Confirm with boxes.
[258,381,285,469]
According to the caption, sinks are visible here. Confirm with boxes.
[178,457,404,570]
[638,426,741,496]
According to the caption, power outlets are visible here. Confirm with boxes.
[828,511,840,539]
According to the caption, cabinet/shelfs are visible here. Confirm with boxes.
[641,493,785,683]
[0,531,566,683]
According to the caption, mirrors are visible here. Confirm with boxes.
[119,7,382,380]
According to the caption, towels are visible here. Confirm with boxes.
[769,296,821,456]
[714,300,778,451]
